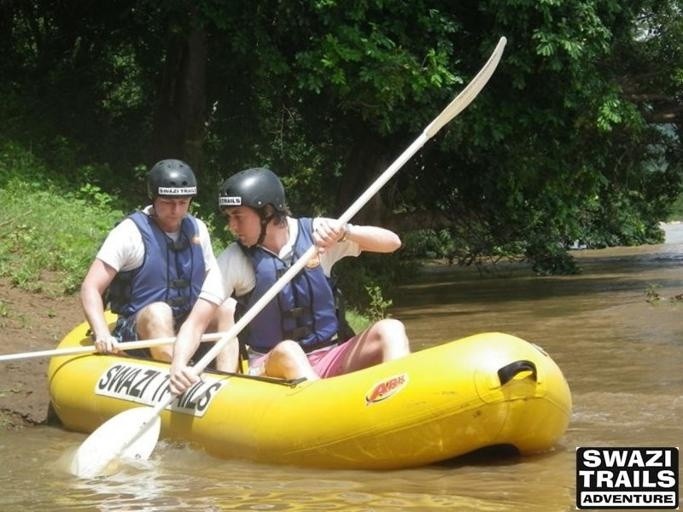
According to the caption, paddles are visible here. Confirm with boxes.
[69,37,507,482]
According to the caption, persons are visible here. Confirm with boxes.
[168,165,411,395]
[80,159,242,375]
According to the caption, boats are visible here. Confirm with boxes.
[46,307,573,473]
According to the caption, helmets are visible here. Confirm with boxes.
[218,168,285,212]
[147,159,196,200]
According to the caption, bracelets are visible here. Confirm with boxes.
[338,222,350,243]
